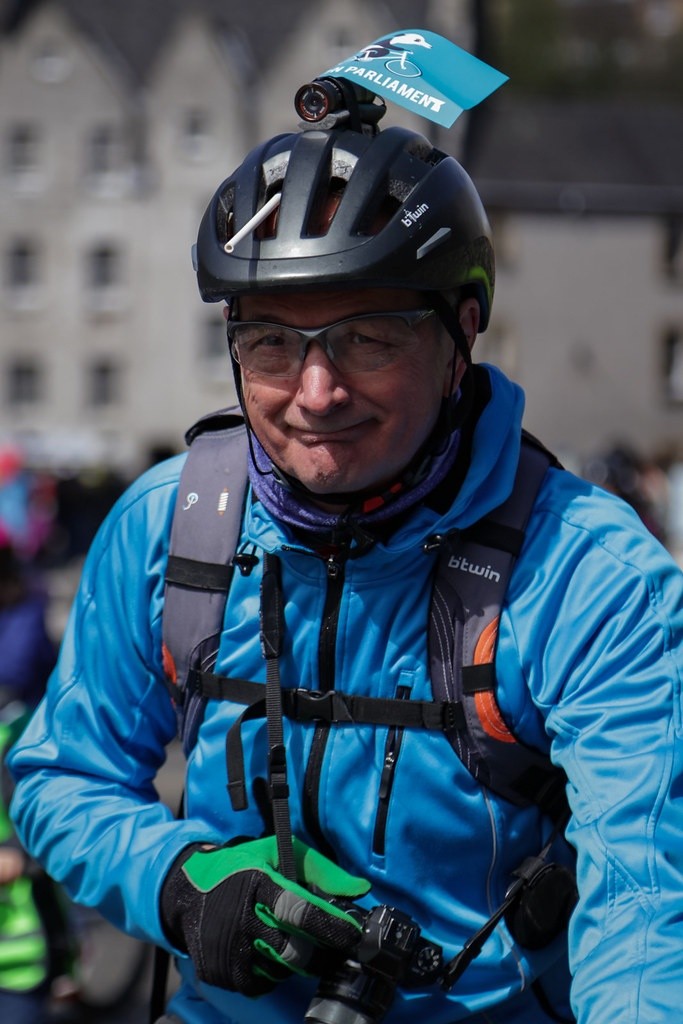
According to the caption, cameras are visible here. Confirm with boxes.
[302,895,444,1024]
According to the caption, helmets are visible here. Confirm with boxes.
[191,117,499,334]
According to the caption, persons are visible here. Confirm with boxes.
[0,473,188,1024]
[0,126,683,1024]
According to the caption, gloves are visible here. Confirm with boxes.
[154,831,378,997]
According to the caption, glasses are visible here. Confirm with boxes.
[221,300,453,377]
